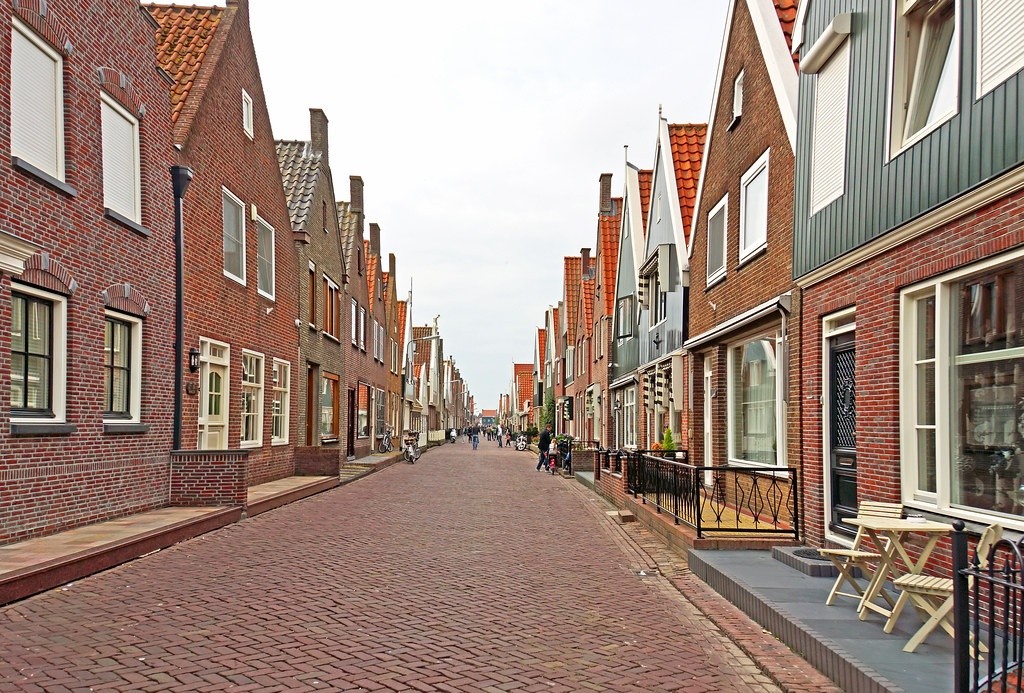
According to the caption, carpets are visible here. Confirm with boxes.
[793,549,832,561]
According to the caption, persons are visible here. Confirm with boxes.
[535,424,558,473]
[460,422,522,450]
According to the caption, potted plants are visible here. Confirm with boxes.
[651,427,675,460]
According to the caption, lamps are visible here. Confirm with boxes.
[189,347,201,373]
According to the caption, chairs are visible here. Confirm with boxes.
[817,501,904,613]
[893,524,1003,661]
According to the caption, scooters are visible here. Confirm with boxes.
[515,434,528,451]
[402,429,422,464]
[542,450,560,474]
[449,429,457,444]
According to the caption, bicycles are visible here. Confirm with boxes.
[377,422,394,453]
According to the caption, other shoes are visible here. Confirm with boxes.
[544,470,551,473]
[536,467,541,473]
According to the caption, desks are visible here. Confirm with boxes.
[842,517,967,634]
